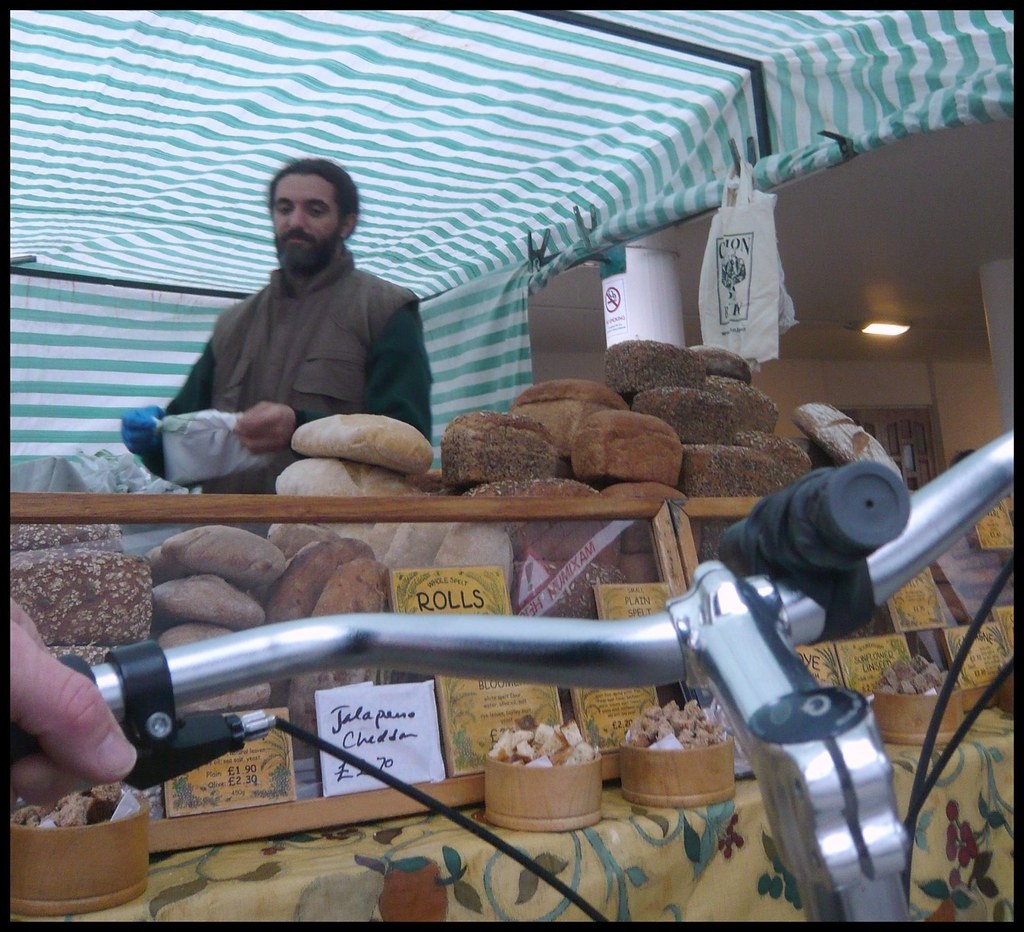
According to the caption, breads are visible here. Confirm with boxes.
[10,340,813,764]
[789,399,1014,626]
[11,781,123,827]
[873,655,955,695]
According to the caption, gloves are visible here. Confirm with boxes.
[121,406,164,455]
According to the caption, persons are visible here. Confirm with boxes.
[121,158,433,536]
